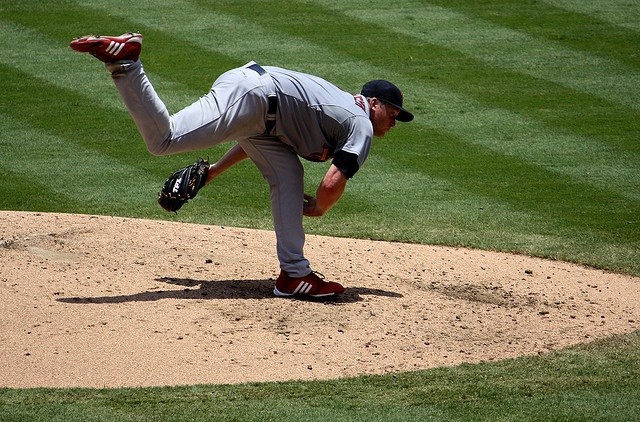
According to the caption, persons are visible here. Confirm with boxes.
[69,32,414,300]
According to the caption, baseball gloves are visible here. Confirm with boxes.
[158,155,210,215]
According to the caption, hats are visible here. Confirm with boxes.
[360,79,414,122]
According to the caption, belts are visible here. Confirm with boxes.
[248,63,278,137]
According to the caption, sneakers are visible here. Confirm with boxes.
[273,268,344,300]
[69,32,143,69]
